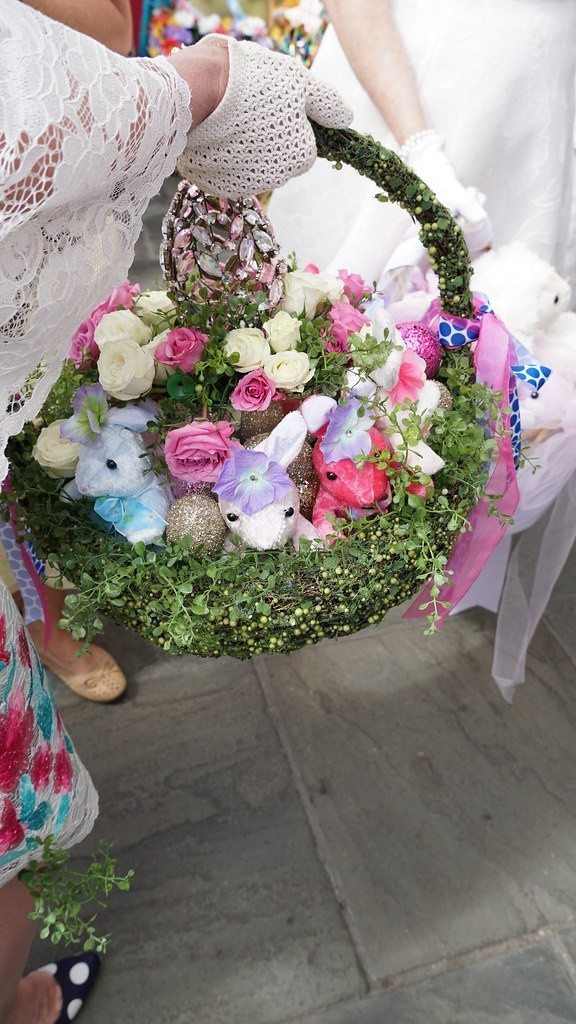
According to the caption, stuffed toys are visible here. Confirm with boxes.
[60,421,168,547]
[471,238,576,431]
[217,411,322,557]
[302,366,435,548]
[342,309,452,480]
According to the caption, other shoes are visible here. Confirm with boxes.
[34,949,101,1024]
[37,642,128,703]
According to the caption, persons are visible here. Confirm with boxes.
[263,1,574,304]
[0,0,470,1024]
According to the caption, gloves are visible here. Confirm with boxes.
[397,129,487,226]
[174,32,354,202]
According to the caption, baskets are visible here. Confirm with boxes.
[366,242,576,537]
[2,120,487,658]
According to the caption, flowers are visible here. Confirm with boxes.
[31,255,428,518]
